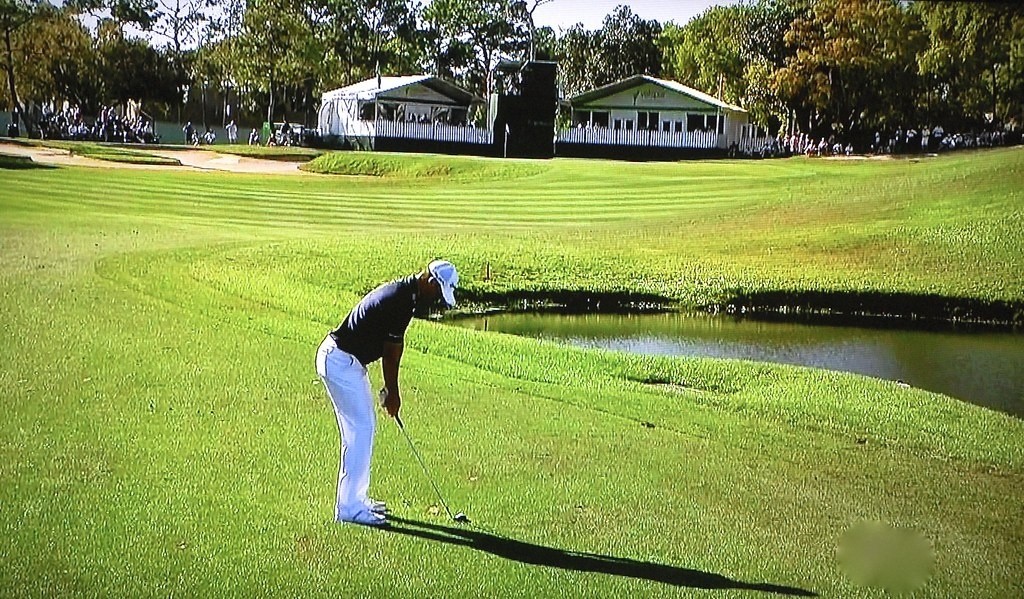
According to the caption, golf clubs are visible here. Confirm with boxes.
[380,389,467,520]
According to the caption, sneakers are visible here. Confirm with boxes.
[335,508,386,526]
[362,496,386,515]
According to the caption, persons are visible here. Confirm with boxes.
[727,122,1024,158]
[314,258,460,526]
[42,104,309,148]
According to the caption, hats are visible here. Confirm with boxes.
[428,261,459,307]
